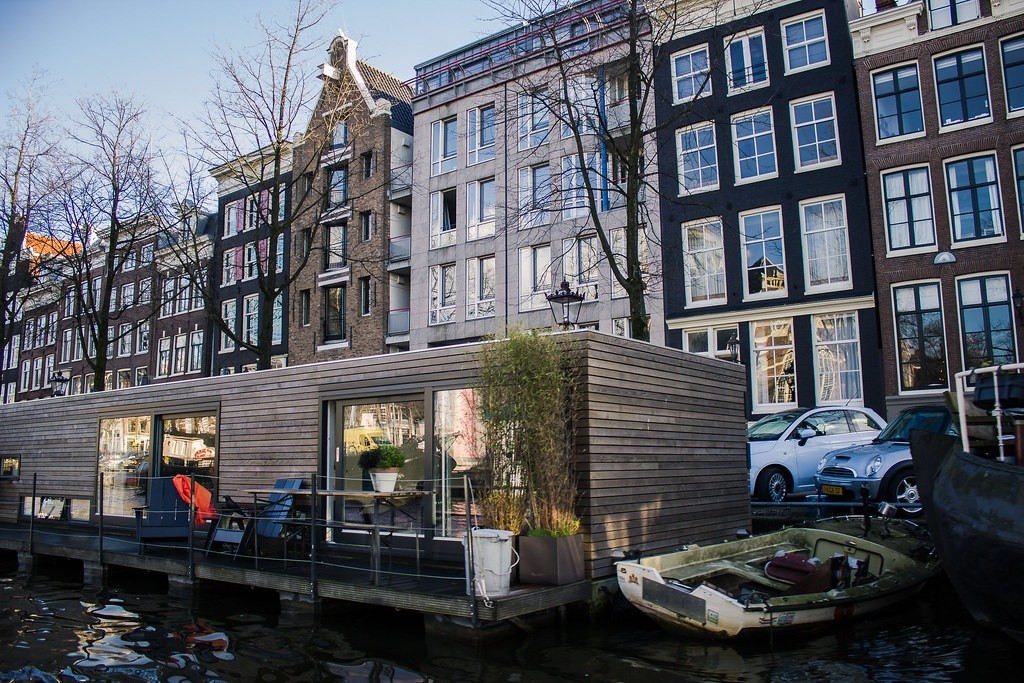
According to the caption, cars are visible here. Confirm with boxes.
[99,448,214,497]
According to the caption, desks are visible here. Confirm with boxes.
[242,488,437,592]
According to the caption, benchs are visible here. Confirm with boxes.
[730,564,789,592]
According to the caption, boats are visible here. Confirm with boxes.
[610,482,945,645]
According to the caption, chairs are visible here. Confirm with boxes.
[205,478,303,562]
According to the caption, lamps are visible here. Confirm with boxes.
[544,278,586,332]
[50,370,71,397]
[726,333,741,364]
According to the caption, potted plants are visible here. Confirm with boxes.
[460,316,586,587]
[356,445,406,492]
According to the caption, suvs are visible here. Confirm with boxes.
[812,401,1017,519]
[747,406,896,503]
